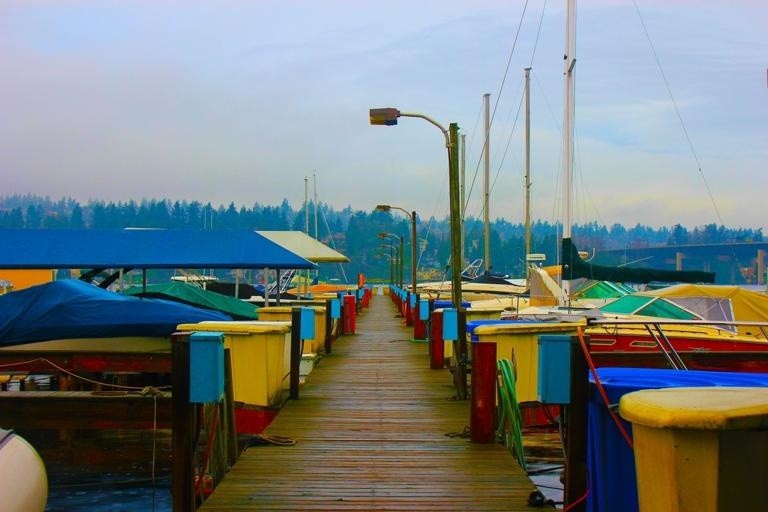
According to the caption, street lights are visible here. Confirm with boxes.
[368,107,469,401]
[377,204,416,295]
